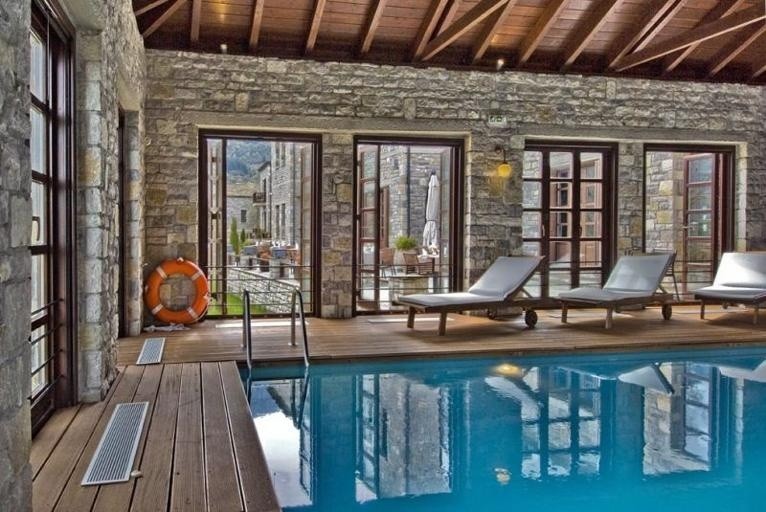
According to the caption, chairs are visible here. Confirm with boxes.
[548,253,683,329]
[391,253,560,336]
[694,252,766,324]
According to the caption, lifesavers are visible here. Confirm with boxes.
[146,259,208,324]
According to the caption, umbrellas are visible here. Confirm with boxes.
[420,168,442,272]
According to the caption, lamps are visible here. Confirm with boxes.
[493,145,511,178]
